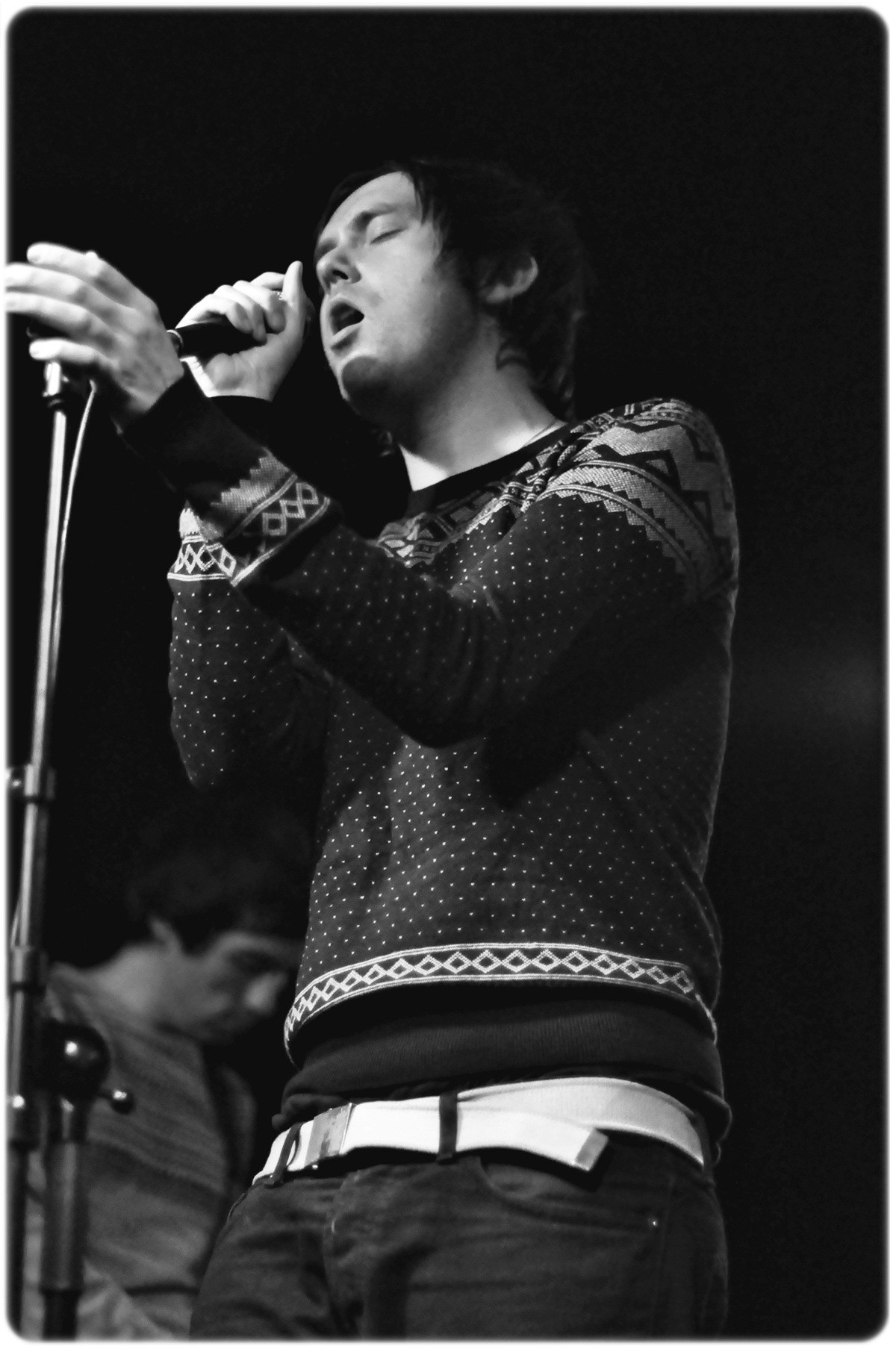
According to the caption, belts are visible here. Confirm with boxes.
[249,1075,706,1185]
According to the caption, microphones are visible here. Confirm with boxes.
[165,291,315,358]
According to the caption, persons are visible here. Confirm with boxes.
[19,834,310,1339]
[6,151,743,1340]
